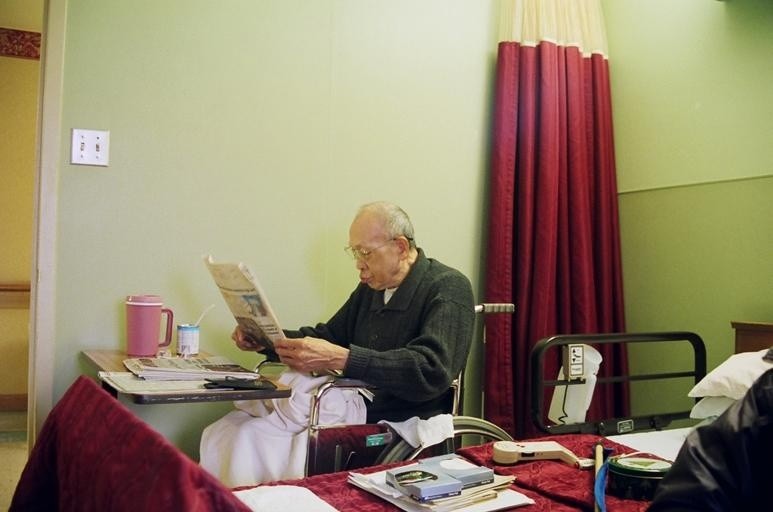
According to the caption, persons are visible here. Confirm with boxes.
[199,201,476,488]
[648,368,772,512]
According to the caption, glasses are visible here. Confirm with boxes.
[344,234,413,261]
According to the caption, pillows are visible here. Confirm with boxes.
[687,347,773,400]
[689,396,736,421]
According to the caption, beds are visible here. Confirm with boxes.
[9,330,773,510]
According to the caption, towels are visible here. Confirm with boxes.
[377,413,455,449]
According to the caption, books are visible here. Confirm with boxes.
[348,463,535,512]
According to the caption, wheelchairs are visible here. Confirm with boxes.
[251,303,516,478]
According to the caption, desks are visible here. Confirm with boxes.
[81,349,292,406]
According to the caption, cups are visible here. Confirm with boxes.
[123,294,173,359]
[176,324,200,358]
